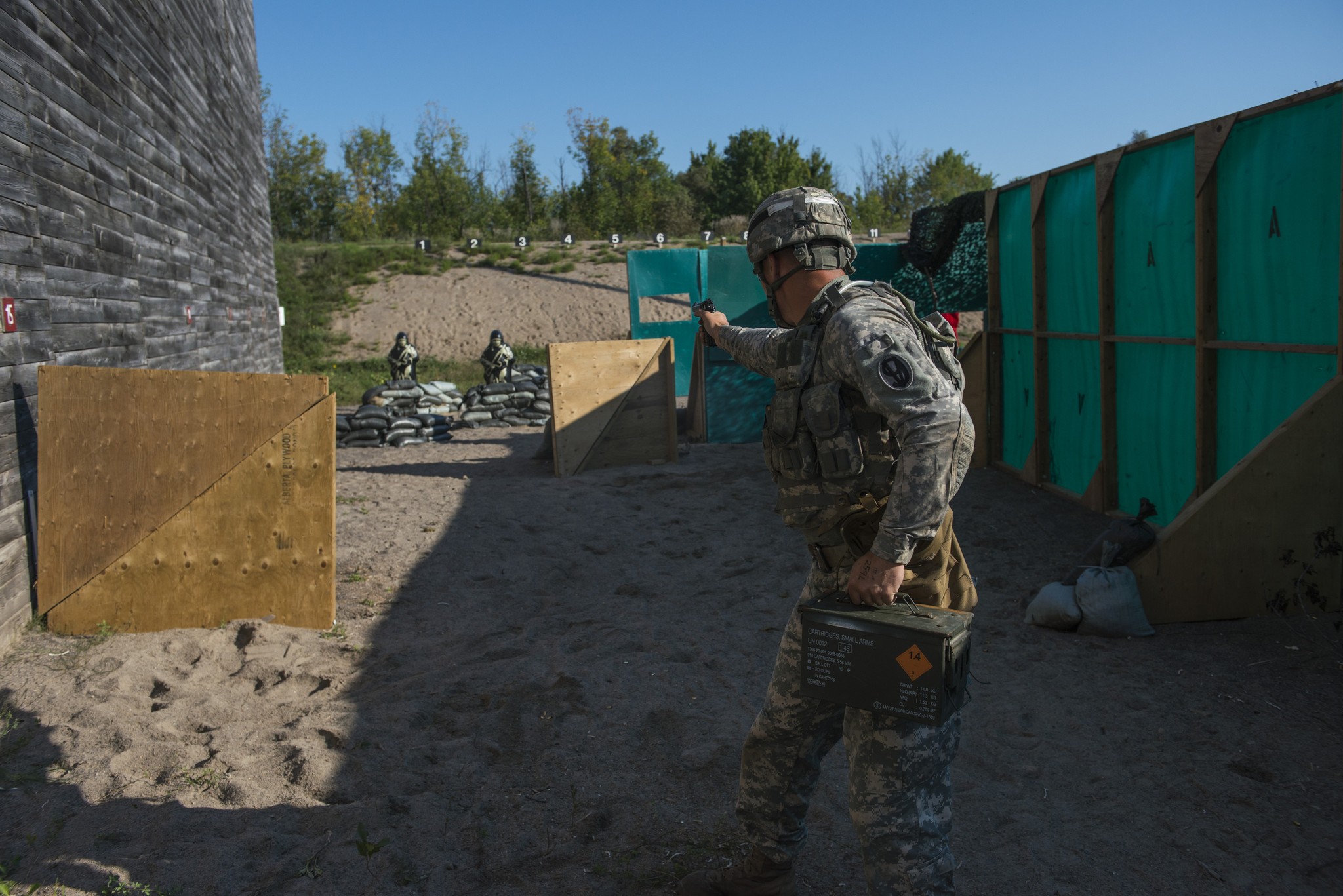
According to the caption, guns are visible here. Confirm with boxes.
[692,298,717,348]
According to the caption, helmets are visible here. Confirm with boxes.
[745,186,858,266]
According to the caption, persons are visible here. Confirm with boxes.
[479,328,517,386]
[689,182,990,895]
[386,330,421,386]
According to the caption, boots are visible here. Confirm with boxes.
[678,843,797,896]
